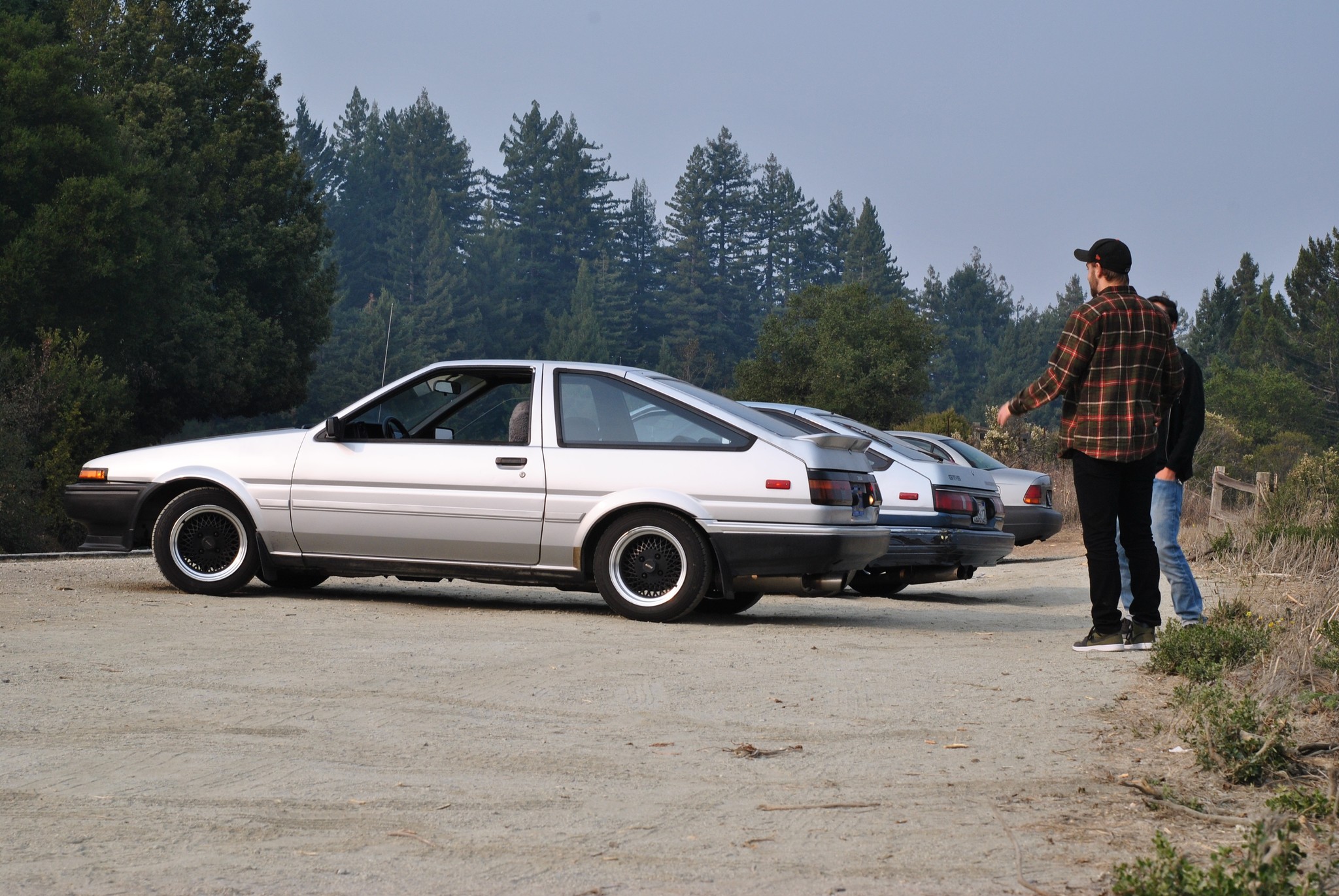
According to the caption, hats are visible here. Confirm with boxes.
[1073,238,1132,274]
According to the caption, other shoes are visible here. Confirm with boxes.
[1180,618,1199,627]
[1121,618,1133,634]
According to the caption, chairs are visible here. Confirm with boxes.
[507,399,529,443]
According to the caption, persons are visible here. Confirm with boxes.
[1116,296,1207,627]
[997,238,1176,652]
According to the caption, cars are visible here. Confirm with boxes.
[65,358,1063,625]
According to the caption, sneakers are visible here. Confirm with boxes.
[1124,622,1156,650]
[1072,626,1124,651]
